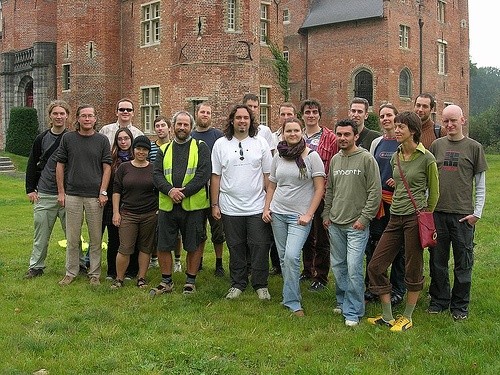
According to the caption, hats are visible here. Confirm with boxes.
[133,135,151,149]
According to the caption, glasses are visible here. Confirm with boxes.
[117,108,133,112]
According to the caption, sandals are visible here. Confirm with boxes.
[110,281,124,291]
[136,279,149,290]
[149,282,175,295]
[183,284,197,295]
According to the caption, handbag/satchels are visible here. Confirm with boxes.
[418,212,438,249]
[36,157,46,172]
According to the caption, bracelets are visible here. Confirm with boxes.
[210,202,219,208]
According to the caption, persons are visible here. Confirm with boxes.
[144,115,184,274]
[209,104,274,302]
[344,96,381,302]
[147,110,213,296]
[241,93,277,277]
[320,118,384,327]
[424,103,490,322]
[261,116,327,317]
[187,100,228,278]
[106,125,141,280]
[366,110,443,333]
[110,134,160,291]
[296,97,341,293]
[84,98,146,267]
[410,92,448,151]
[268,100,299,277]
[25,98,90,280]
[55,102,114,286]
[364,103,407,306]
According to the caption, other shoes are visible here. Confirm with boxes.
[332,309,342,313]
[199,268,204,273]
[345,319,358,326]
[59,276,76,286]
[124,276,131,280]
[79,267,89,276]
[389,295,404,305]
[364,290,379,301]
[90,277,99,287]
[216,268,225,276]
[106,277,114,280]
[295,310,305,318]
[24,269,43,279]
[268,267,279,274]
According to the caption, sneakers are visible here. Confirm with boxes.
[257,288,270,301]
[298,274,317,281]
[367,315,395,327]
[226,288,243,300]
[147,263,160,271]
[390,317,414,332]
[428,303,449,314]
[307,282,327,292]
[452,308,469,320]
[175,264,182,274]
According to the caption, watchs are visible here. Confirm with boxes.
[98,190,108,195]
